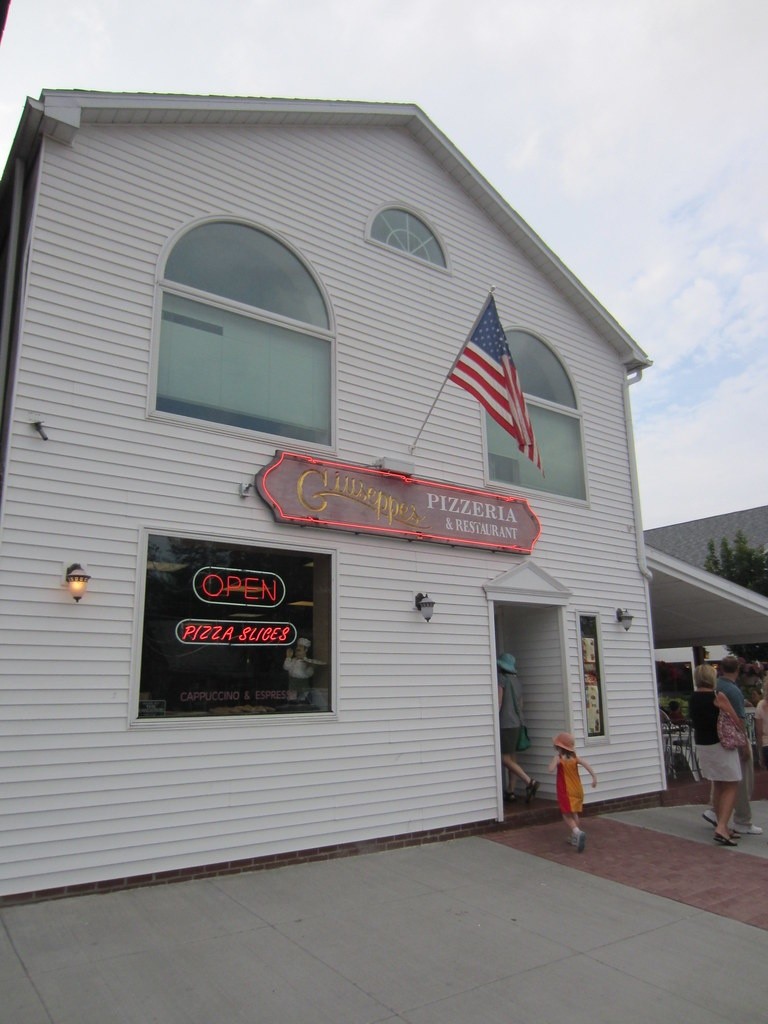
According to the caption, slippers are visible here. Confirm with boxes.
[712,835,737,846]
[503,790,517,804]
[715,832,741,839]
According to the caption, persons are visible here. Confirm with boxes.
[549,732,597,853]
[496,652,540,806]
[667,701,684,720]
[689,656,768,846]
[283,637,314,705]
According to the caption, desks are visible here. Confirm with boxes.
[665,722,698,772]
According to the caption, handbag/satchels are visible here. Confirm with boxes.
[516,725,532,752]
[714,689,747,750]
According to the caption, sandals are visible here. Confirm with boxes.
[526,779,540,804]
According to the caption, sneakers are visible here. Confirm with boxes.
[576,831,586,853]
[702,809,718,827]
[565,835,577,846]
[732,823,764,835]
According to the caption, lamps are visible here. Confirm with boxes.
[378,458,414,475]
[67,562,90,603]
[616,607,633,632]
[415,592,437,623]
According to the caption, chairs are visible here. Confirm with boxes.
[659,708,702,784]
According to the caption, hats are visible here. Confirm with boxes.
[497,653,517,673]
[552,732,576,752]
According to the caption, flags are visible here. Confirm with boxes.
[448,293,545,478]
